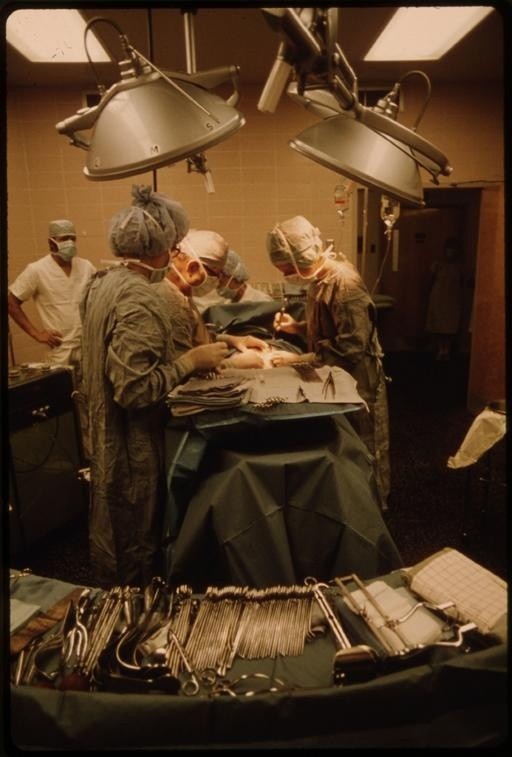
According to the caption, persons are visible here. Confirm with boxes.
[167,228,271,370]
[8,217,99,366]
[71,180,230,591]
[215,249,274,306]
[263,212,394,513]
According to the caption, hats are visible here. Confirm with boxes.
[176,228,248,281]
[266,215,322,266]
[48,219,76,238]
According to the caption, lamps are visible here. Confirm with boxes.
[58,8,242,193]
[260,8,454,208]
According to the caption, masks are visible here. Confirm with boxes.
[190,276,238,300]
[57,240,77,262]
[282,272,316,287]
[148,255,171,283]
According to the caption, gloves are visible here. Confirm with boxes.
[174,312,316,370]
